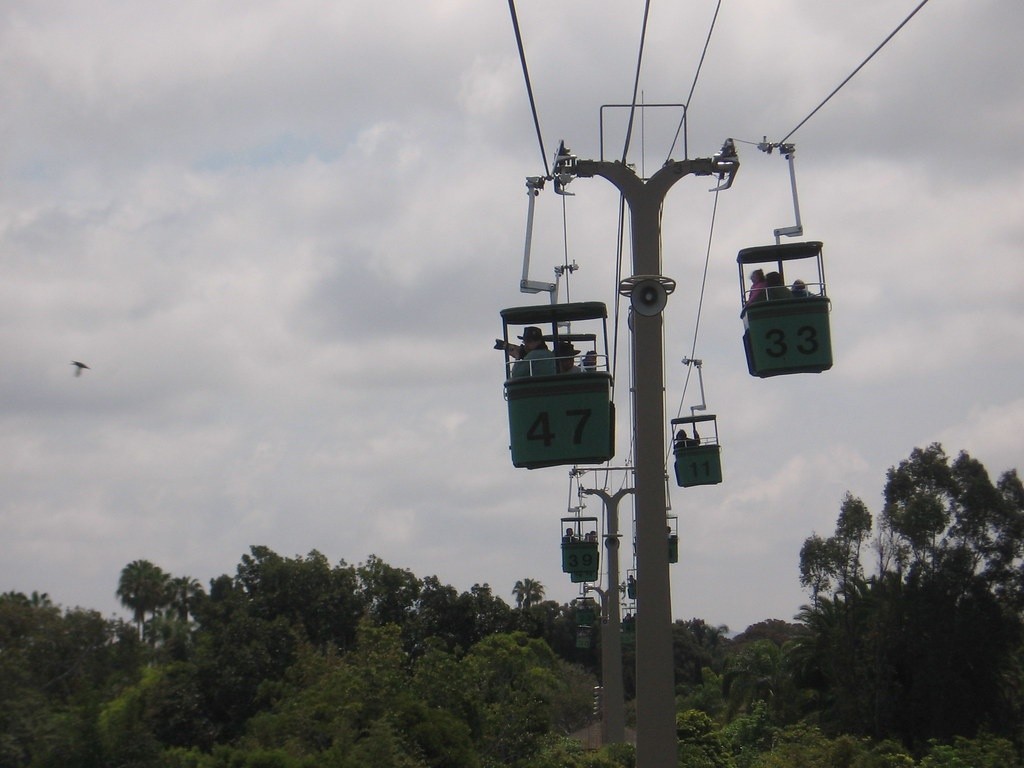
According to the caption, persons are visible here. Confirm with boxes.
[624,613,637,622]
[563,528,596,542]
[674,429,701,448]
[494,326,597,378]
[741,270,814,318]
[626,575,636,582]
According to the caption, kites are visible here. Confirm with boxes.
[71,361,89,378]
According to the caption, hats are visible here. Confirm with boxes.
[551,343,581,357]
[792,281,806,290]
[766,271,784,289]
[517,326,548,340]
